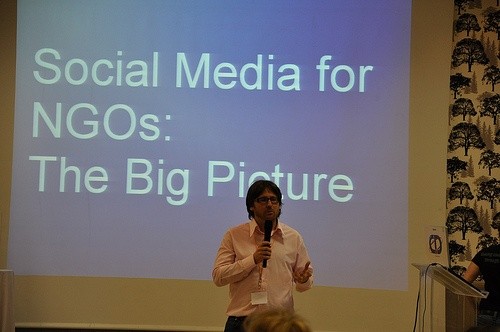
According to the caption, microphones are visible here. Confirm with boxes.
[262,219,273,268]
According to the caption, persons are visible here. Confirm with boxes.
[242,304,312,331]
[463,243,500,332]
[212,180,313,332]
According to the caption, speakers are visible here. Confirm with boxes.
[0,270,16,332]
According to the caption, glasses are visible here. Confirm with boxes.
[253,197,280,204]
